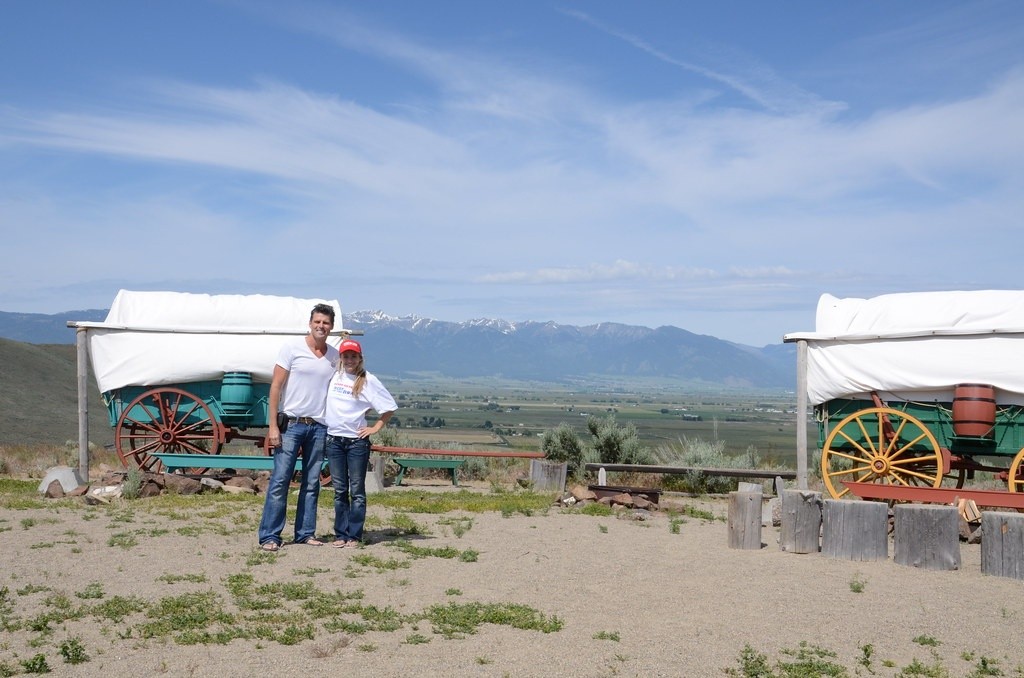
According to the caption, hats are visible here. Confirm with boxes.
[339,340,361,354]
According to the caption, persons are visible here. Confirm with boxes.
[325,339,399,549]
[257,303,371,551]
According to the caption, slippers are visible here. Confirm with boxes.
[333,541,345,548]
[262,542,278,551]
[344,540,358,549]
[295,538,323,547]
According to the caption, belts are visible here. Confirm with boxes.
[288,417,315,423]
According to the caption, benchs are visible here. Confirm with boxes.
[584,462,796,496]
[392,458,465,487]
